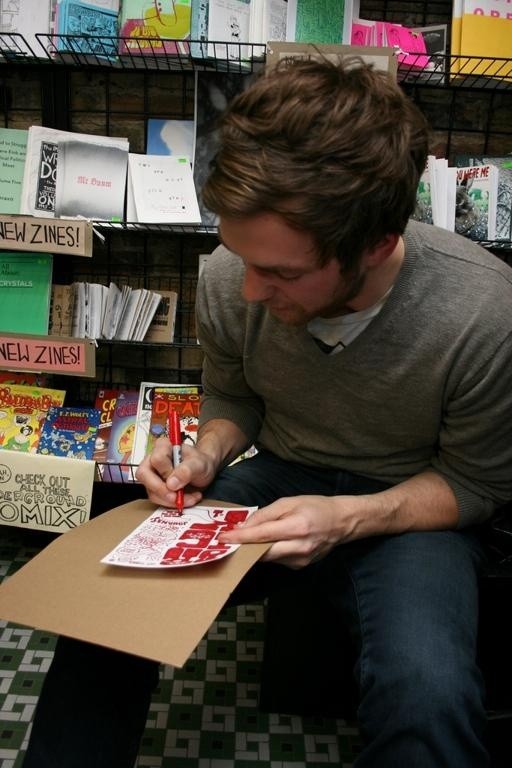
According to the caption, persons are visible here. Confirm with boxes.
[22,42,512,767]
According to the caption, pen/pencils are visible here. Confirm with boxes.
[169,409,184,517]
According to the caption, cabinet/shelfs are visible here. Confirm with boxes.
[2,26,512,519]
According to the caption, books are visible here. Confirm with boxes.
[2,0,511,90]
[409,154,511,249]
[1,123,220,233]
[1,251,178,345]
[0,369,258,486]
